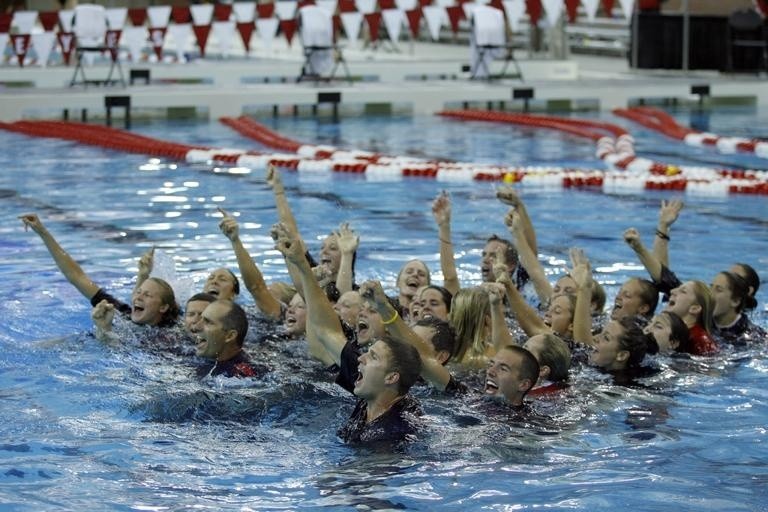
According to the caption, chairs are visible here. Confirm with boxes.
[470,7,525,84]
[296,8,353,87]
[726,8,768,77]
[70,6,126,89]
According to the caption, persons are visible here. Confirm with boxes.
[17,162,768,446]
